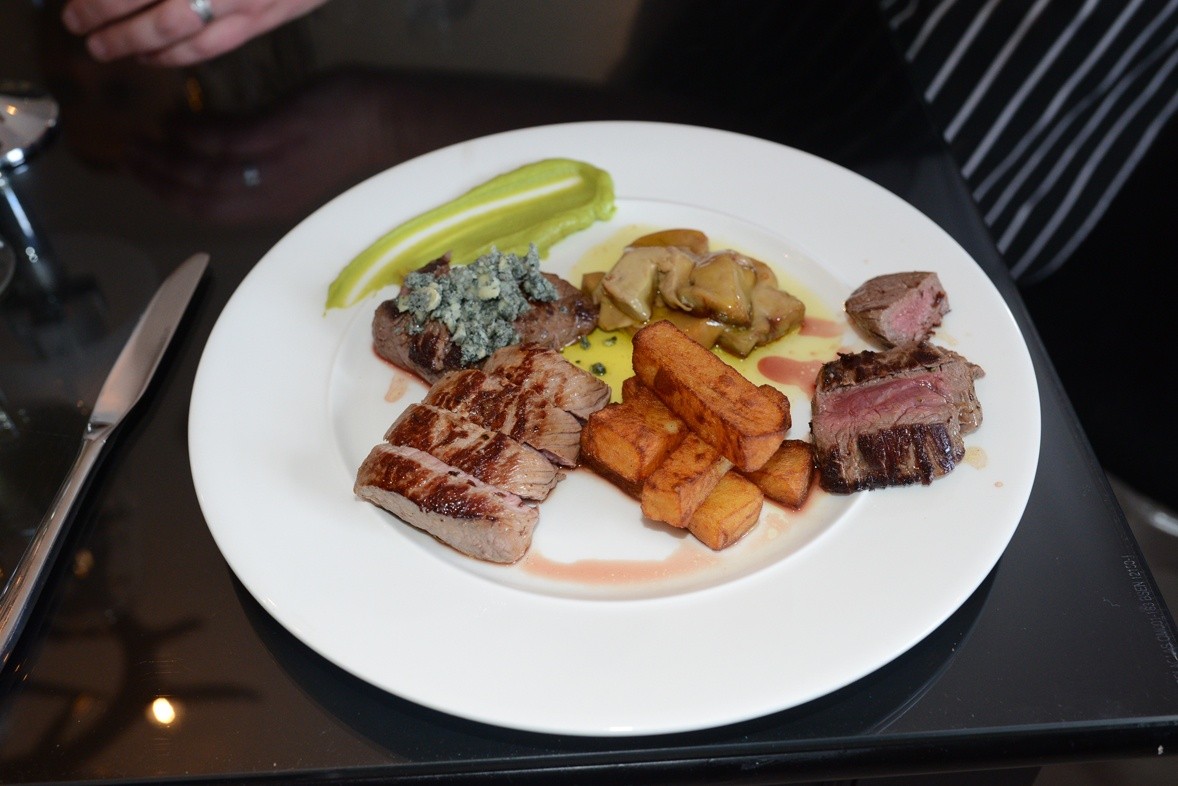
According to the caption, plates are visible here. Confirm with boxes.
[189,118,1042,735]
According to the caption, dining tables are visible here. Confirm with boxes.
[0,0,1178,786]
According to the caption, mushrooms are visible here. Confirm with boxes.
[594,246,806,357]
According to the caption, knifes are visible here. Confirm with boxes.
[0,251,205,671]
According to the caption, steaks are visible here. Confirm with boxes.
[809,270,985,495]
[354,251,613,564]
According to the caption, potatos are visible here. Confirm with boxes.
[578,319,815,551]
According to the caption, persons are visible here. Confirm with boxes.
[61,1,331,68]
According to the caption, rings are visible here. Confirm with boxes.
[187,0,213,23]
